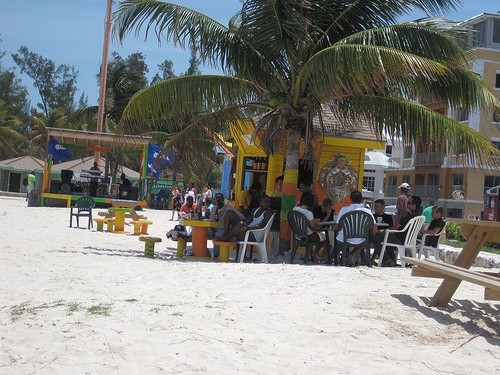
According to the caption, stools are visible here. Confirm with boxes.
[138,236,162,258]
[93,217,115,232]
[171,235,192,258]
[211,240,236,261]
[131,218,153,236]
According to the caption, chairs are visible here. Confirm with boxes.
[237,212,277,263]
[334,209,374,267]
[416,221,448,261]
[70,196,96,230]
[287,209,328,264]
[377,214,426,267]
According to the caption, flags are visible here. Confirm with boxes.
[48,136,72,160]
[146,144,174,177]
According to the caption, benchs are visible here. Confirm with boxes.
[400,256,500,291]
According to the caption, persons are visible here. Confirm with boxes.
[268,175,283,197]
[169,181,212,221]
[395,182,411,224]
[89,162,100,197]
[292,192,329,261]
[332,190,377,267]
[234,181,262,210]
[313,198,336,264]
[27,171,35,194]
[421,197,435,230]
[118,173,132,194]
[204,192,245,240]
[374,196,423,266]
[373,200,398,267]
[416,205,446,247]
[215,195,274,242]
[297,181,306,190]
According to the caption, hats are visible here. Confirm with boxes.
[174,226,184,231]
[397,183,409,189]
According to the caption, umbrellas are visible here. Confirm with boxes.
[0,156,49,173]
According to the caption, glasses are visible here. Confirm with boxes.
[261,200,271,204]
[407,201,413,204]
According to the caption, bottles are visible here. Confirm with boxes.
[253,157,267,170]
[191,208,210,221]
[215,209,219,222]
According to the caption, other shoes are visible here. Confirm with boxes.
[383,261,394,266]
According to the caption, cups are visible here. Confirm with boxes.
[334,212,338,221]
[377,217,383,223]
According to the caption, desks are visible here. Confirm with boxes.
[108,207,132,232]
[182,219,220,260]
[428,217,500,309]
[318,220,389,268]
